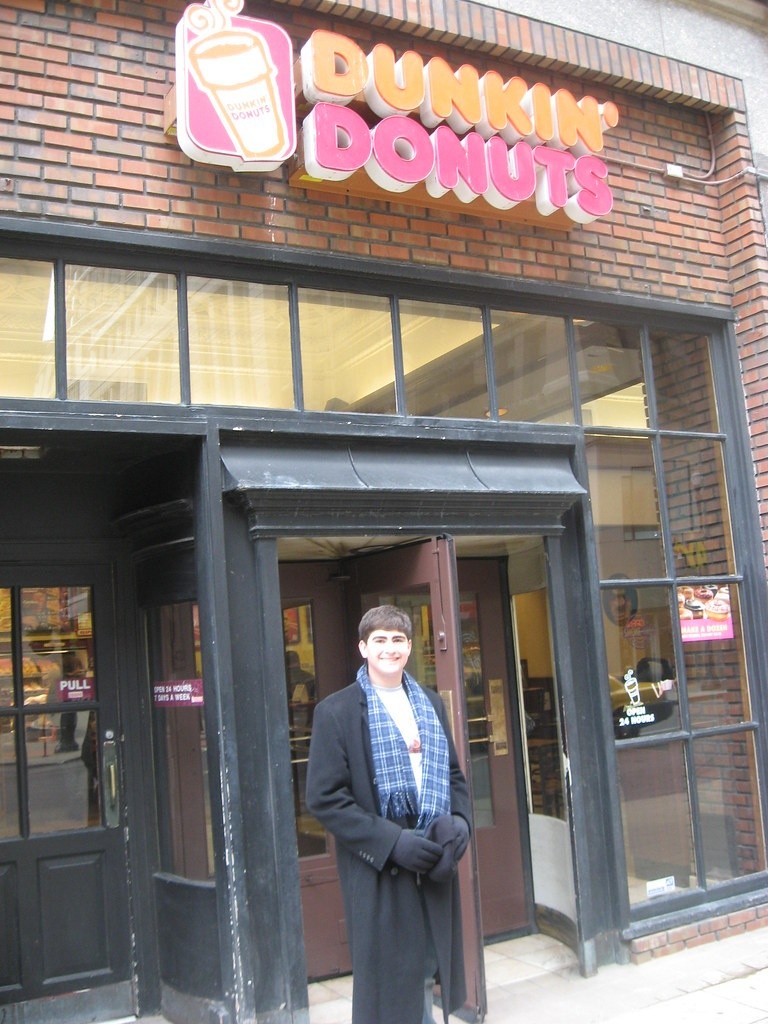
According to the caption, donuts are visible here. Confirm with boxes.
[675,585,731,621]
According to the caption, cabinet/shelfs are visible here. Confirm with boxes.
[0,673,52,733]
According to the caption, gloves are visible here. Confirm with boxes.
[453,813,470,862]
[389,827,442,875]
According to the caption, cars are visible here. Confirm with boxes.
[606,673,673,741]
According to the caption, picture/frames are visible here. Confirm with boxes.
[306,605,313,644]
[284,608,301,646]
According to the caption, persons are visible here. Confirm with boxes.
[286,651,314,698]
[304,604,474,1024]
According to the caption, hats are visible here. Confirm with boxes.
[420,815,458,883]
